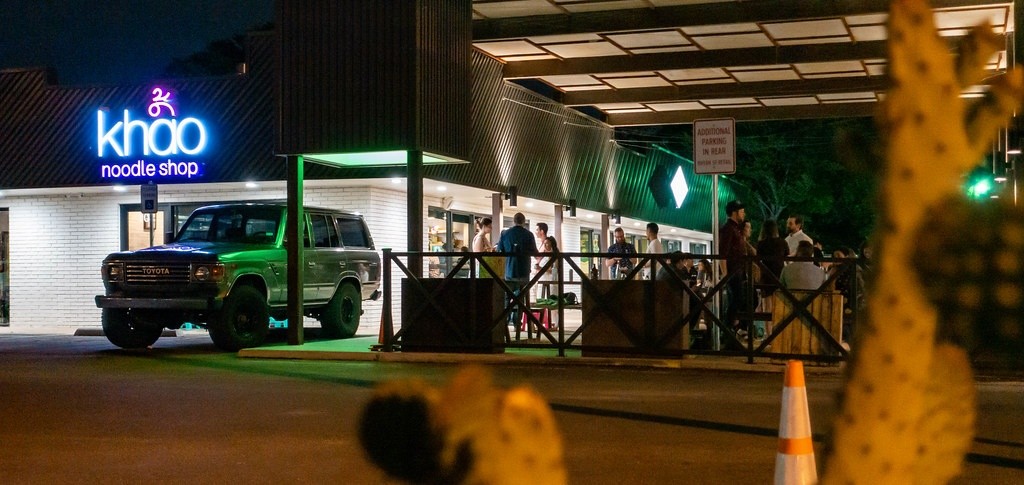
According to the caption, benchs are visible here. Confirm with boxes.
[733,312,773,321]
[505,302,582,313]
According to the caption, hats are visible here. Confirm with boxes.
[726,197,751,215]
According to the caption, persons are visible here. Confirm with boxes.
[605,228,637,280]
[473,217,496,278]
[657,250,713,328]
[429,232,470,278]
[719,201,871,352]
[536,223,559,331]
[495,212,539,331]
[643,223,663,280]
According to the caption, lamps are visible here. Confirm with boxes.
[611,209,621,224]
[442,197,454,210]
[505,185,517,209]
[566,199,576,216]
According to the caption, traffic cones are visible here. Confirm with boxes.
[773,361,820,485]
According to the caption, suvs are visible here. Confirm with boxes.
[95,201,382,353]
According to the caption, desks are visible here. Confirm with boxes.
[504,279,533,341]
[537,280,582,344]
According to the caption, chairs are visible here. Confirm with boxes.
[225,226,246,242]
